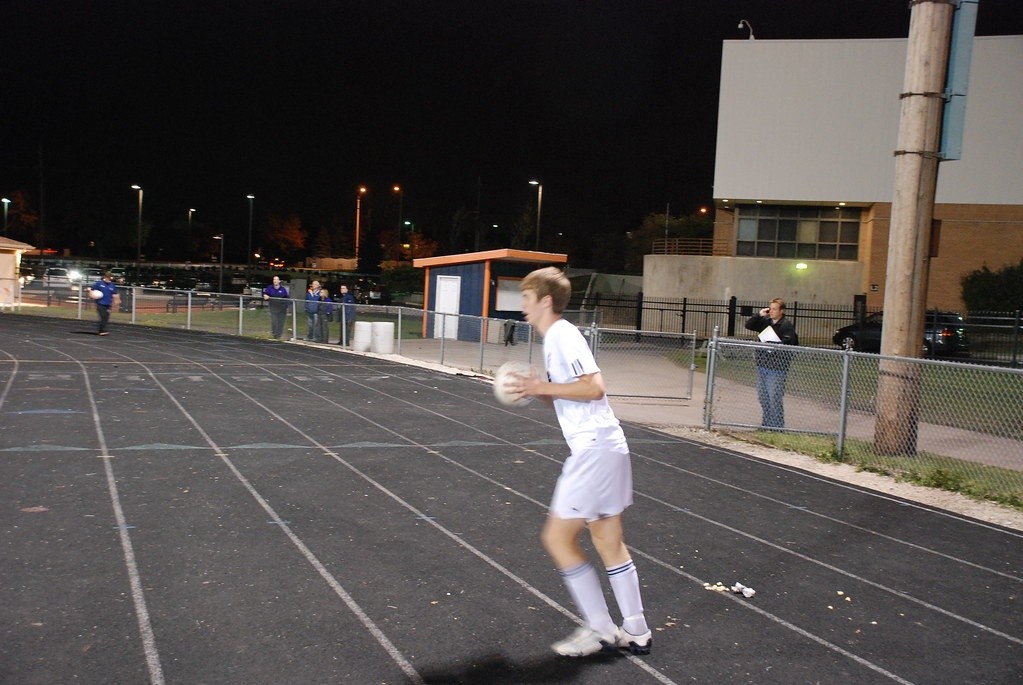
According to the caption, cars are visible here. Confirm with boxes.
[268,257,287,272]
[331,275,392,306]
[142,277,177,296]
[833,307,970,359]
[19,265,127,291]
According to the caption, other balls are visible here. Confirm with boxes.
[492,360,540,408]
[89,290,104,300]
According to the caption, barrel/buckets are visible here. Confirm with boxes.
[353,321,372,351]
[372,322,394,354]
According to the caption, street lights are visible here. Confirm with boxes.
[353,186,366,276]
[528,179,543,251]
[392,185,402,268]
[211,232,224,311]
[185,208,196,264]
[242,194,256,295]
[131,183,144,289]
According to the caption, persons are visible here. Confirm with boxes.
[505,267,652,659]
[304,280,322,341]
[317,289,333,343]
[87,270,115,335]
[746,297,798,433]
[262,275,289,339]
[336,284,356,346]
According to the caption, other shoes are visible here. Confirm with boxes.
[99,331,109,336]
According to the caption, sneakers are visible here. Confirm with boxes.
[553,623,618,659]
[616,626,653,655]
